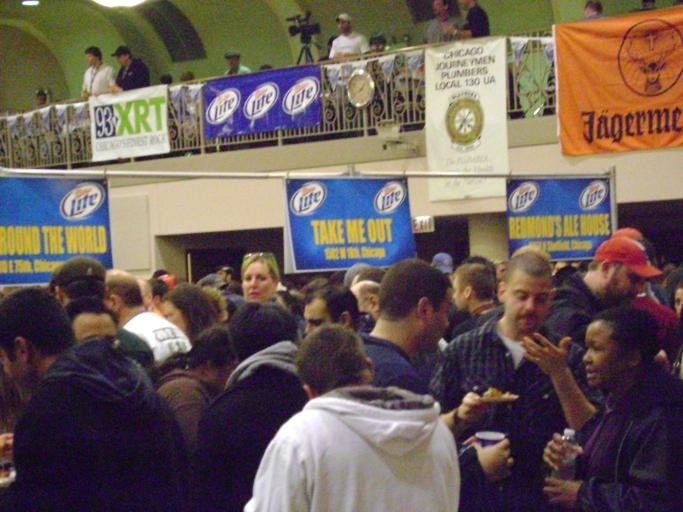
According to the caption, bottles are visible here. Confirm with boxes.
[546,428,577,499]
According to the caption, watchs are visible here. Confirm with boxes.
[453,412,479,436]
[450,29,460,38]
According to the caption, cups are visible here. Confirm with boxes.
[474,431,506,446]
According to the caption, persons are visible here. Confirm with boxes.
[450,262,503,334]
[422,0,459,42]
[241,320,460,511]
[357,256,515,494]
[222,49,252,76]
[495,259,508,279]
[218,266,242,294]
[583,0,603,19]
[542,305,683,511]
[370,36,387,53]
[0,286,193,511]
[156,327,238,447]
[51,253,106,304]
[34,93,48,109]
[110,46,150,93]
[302,284,356,332]
[445,0,490,37]
[237,252,280,303]
[79,46,116,97]
[201,300,299,485]
[329,14,371,58]
[669,277,683,376]
[431,245,604,512]
[350,281,382,317]
[159,280,214,332]
[546,237,663,344]
[349,268,384,284]
[63,297,122,346]
[102,269,192,365]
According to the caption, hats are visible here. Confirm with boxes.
[111,46,129,57]
[335,13,352,21]
[593,236,664,280]
[433,252,453,274]
[223,49,241,58]
[197,273,228,290]
[48,255,104,291]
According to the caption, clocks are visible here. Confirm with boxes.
[347,69,375,110]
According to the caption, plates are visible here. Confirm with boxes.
[480,396,520,403]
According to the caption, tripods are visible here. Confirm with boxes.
[293,43,320,65]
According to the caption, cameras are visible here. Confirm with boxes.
[283,9,323,42]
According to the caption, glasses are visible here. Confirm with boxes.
[242,251,273,260]
